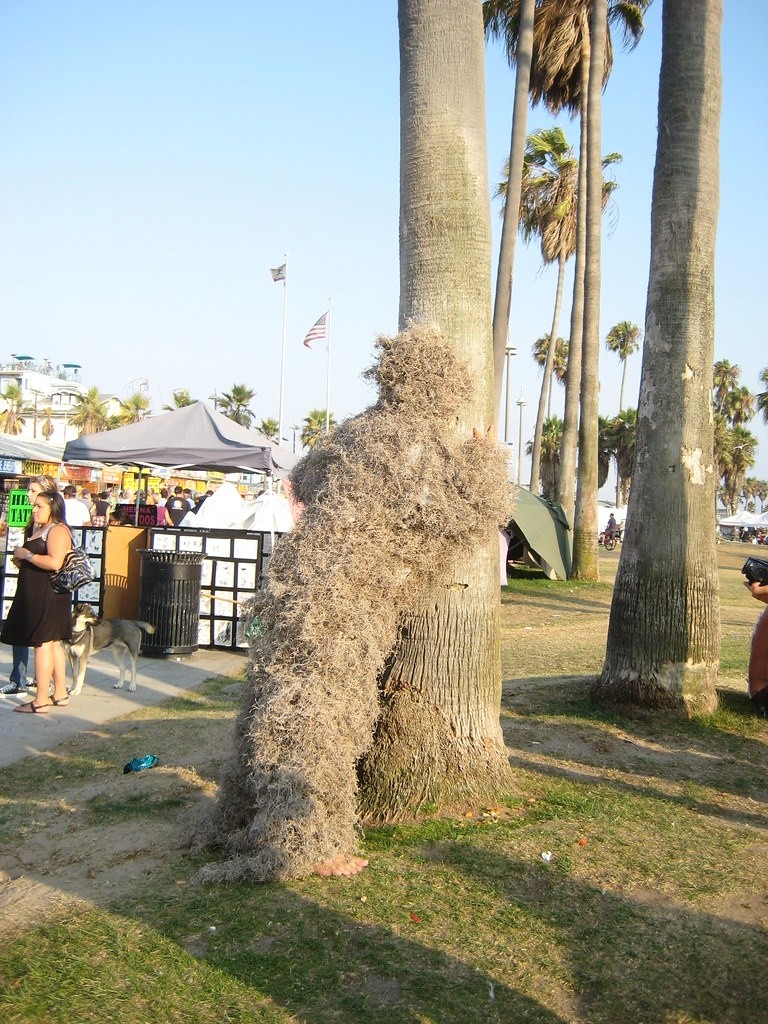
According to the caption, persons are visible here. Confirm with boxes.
[56,481,266,526]
[743,580,768,717]
[11,491,74,713]
[0,474,58,699]
[600,514,616,546]
[739,527,768,546]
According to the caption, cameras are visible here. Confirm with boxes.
[741,556,768,586]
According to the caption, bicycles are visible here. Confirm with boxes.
[598,532,617,551]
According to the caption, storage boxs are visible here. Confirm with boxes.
[7,489,34,527]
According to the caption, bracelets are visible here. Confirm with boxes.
[27,552,34,562]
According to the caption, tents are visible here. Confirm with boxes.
[57,401,300,554]
[509,482,571,581]
[719,510,768,541]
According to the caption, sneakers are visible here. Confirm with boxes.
[25,676,52,691]
[0,681,27,699]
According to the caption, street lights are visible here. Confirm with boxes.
[504,343,518,443]
[515,388,529,487]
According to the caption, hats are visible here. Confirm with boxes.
[101,492,107,499]
[183,489,191,493]
[175,487,184,493]
[83,489,91,494]
[60,485,76,496]
[206,490,215,495]
[161,490,167,494]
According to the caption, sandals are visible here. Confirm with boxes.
[47,694,70,706]
[13,700,49,713]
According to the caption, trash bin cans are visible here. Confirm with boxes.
[136,548,208,660]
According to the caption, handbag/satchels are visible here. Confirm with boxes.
[47,546,97,594]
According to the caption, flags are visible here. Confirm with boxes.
[304,311,328,349]
[271,264,286,281]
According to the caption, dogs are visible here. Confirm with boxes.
[63,603,155,696]
